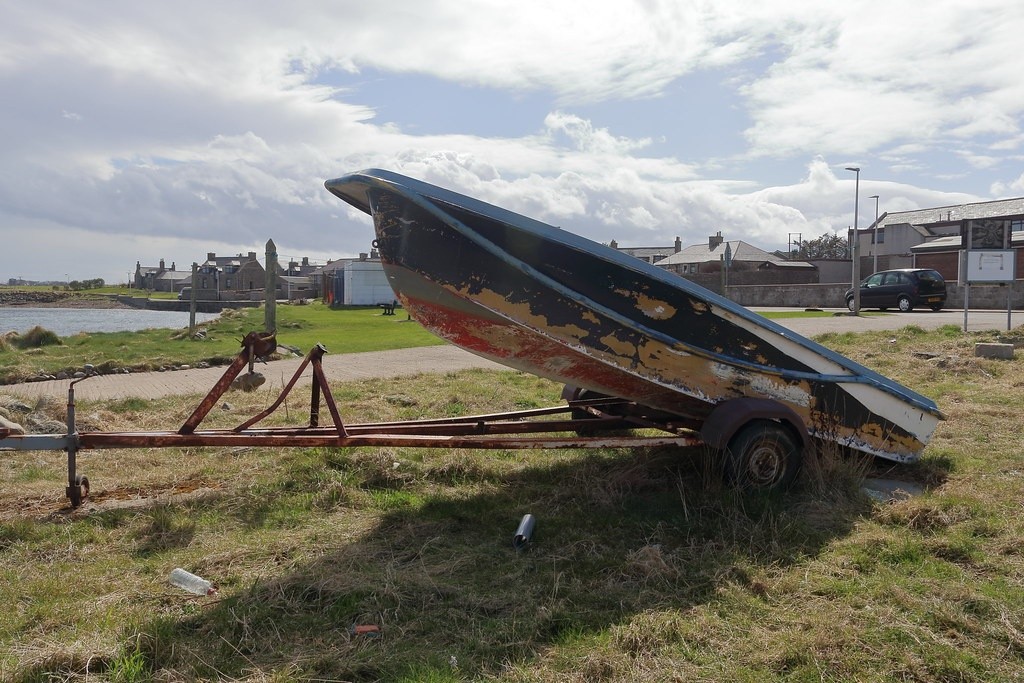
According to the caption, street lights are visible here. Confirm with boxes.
[65,273,69,284]
[843,167,861,315]
[868,195,880,275]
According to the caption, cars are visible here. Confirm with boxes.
[843,268,947,312]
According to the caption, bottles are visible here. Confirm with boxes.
[168,568,215,596]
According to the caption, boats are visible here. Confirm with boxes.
[323,166,950,466]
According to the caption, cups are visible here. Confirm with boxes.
[514,514,535,546]
